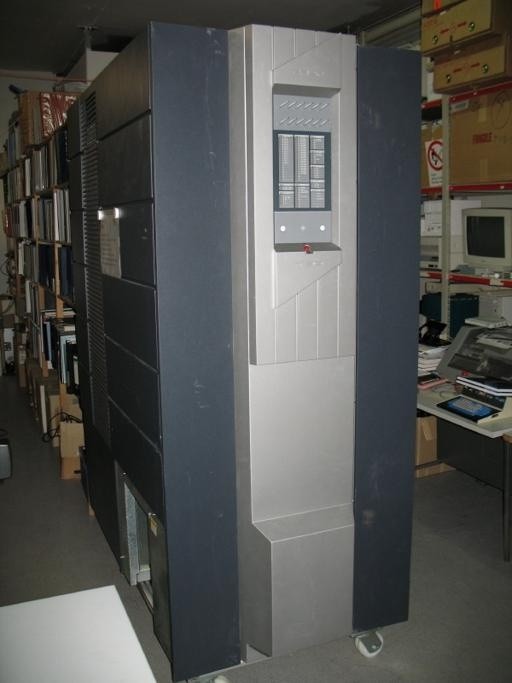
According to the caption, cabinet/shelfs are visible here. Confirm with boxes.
[0,0,512,683]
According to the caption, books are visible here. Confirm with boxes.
[418,316,512,396]
[1,87,82,449]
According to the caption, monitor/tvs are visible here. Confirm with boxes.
[461,207,512,279]
